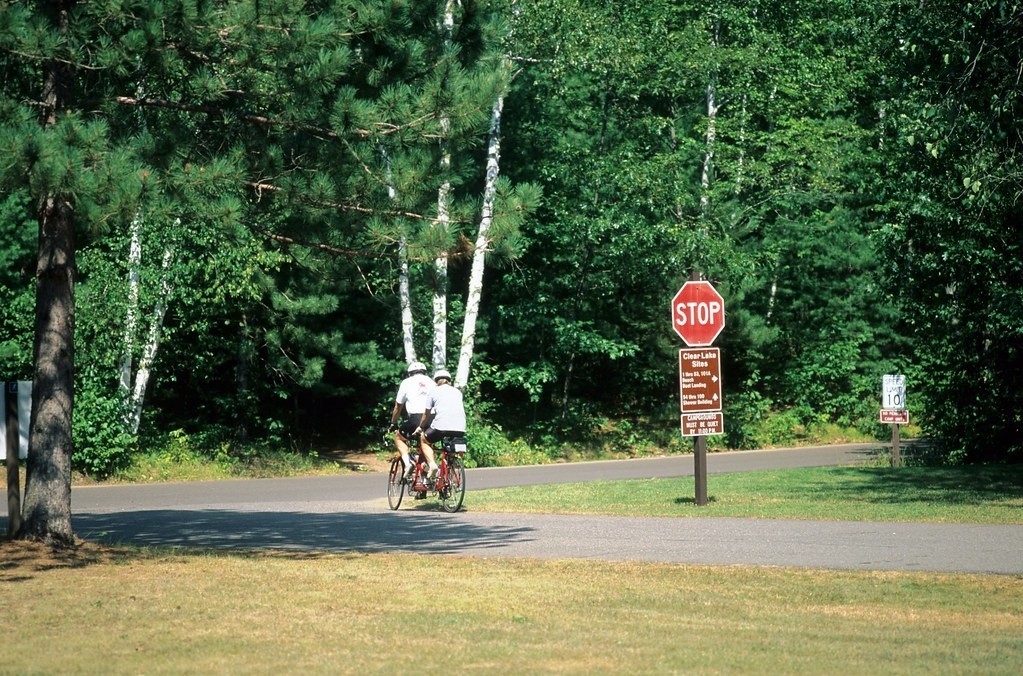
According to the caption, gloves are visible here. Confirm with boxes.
[412,426,423,436]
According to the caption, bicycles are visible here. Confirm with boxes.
[385,424,466,514]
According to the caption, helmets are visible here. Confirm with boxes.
[408,362,426,373]
[433,370,451,382]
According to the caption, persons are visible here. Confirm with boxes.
[387,362,438,499]
[412,371,467,492]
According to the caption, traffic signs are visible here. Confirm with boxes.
[677,348,723,414]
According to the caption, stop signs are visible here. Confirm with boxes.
[670,280,726,347]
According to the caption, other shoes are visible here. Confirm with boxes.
[415,489,427,500]
[403,460,415,478]
[427,465,438,478]
[438,492,450,500]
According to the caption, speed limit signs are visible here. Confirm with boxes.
[882,374,907,411]
